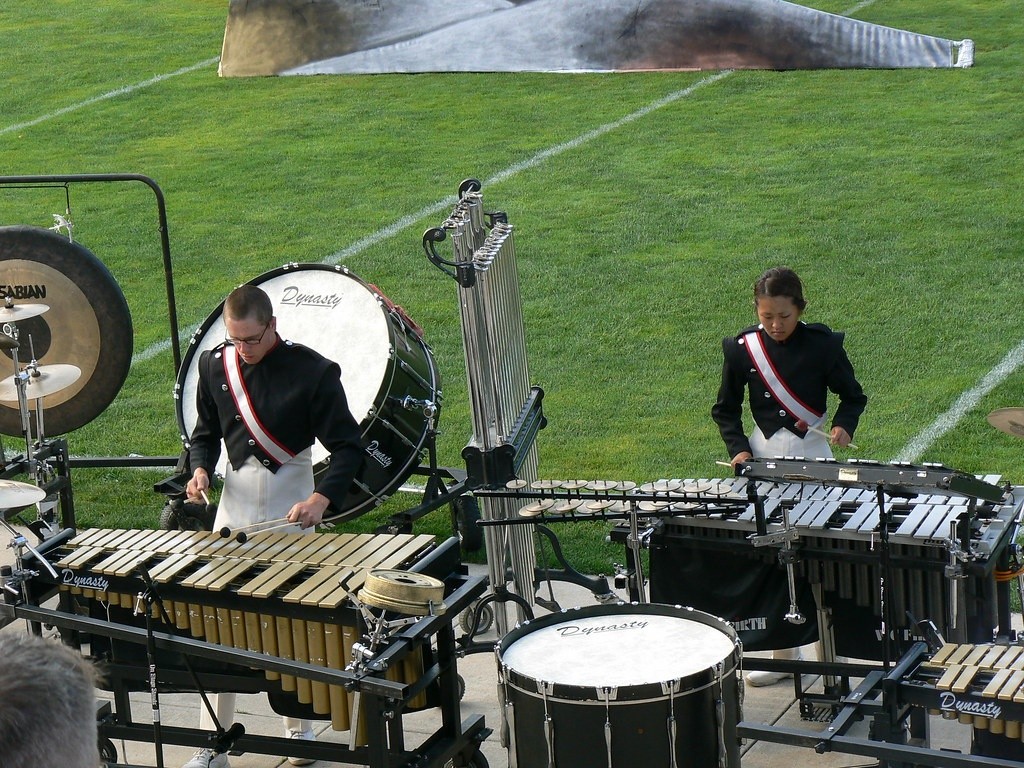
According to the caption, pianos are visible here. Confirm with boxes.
[740,455,1006,506]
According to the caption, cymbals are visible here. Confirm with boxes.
[507,479,740,518]
[0,303,51,323]
[0,363,82,402]
[0,478,47,509]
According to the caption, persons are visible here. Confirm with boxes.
[180,286,365,768]
[712,268,867,687]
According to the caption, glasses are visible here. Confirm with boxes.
[225,320,268,346]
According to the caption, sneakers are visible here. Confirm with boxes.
[285,726,317,765]
[180,747,231,768]
[745,655,807,687]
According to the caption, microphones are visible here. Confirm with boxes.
[209,722,246,759]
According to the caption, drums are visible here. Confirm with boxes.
[492,602,746,768]
[172,260,445,527]
